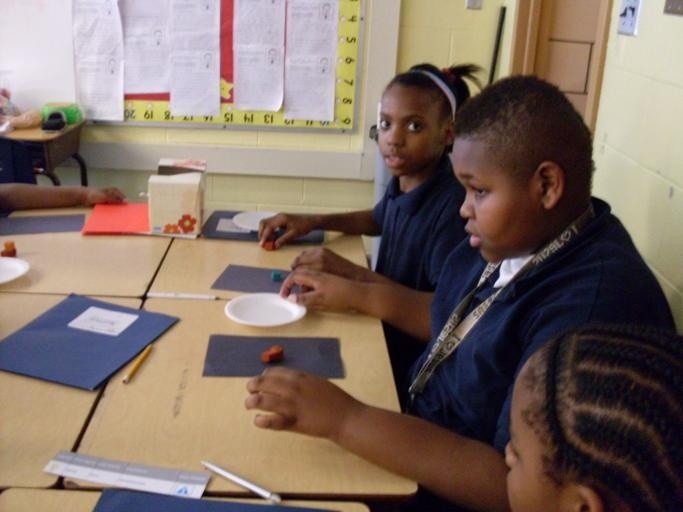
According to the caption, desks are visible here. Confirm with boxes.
[0,207,418,509]
[1,122,90,188]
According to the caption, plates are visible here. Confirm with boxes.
[0,256,30,285]
[224,292,307,329]
[232,210,282,233]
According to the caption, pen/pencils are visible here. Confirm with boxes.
[148,292,218,300]
[123,344,153,385]
[199,459,282,506]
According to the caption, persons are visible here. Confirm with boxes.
[256,63,487,364]
[242,73,681,510]
[0,181,127,216]
[503,317,683,512]
